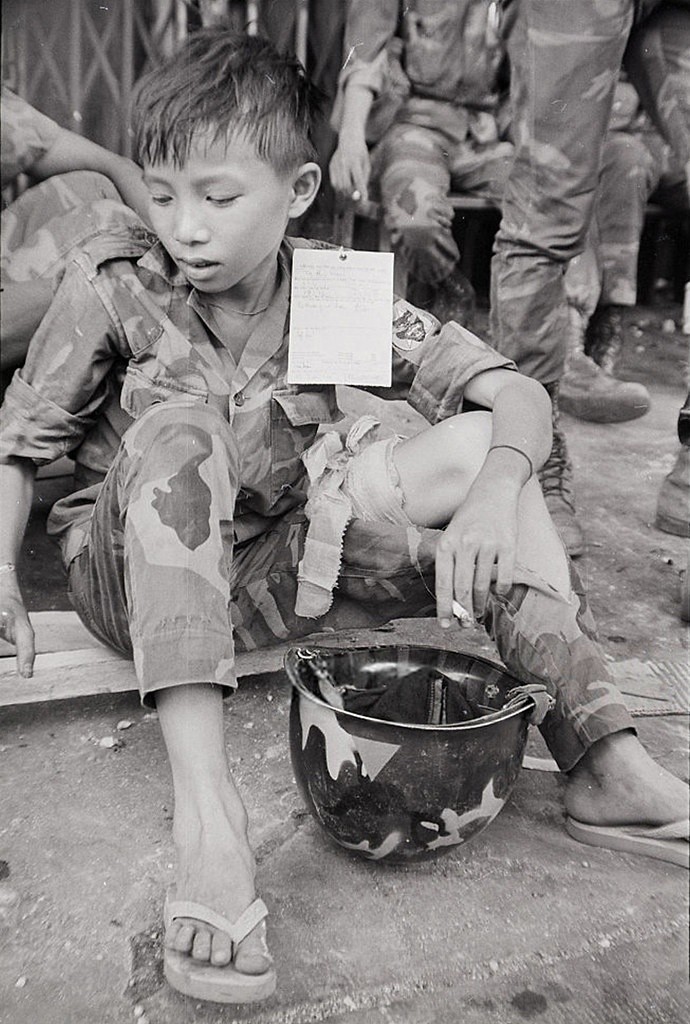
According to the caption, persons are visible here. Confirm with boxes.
[1,26,690,1003]
[330,0,690,557]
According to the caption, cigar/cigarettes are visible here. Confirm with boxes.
[451,594,471,627]
[349,188,361,204]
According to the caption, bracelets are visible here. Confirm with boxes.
[488,445,532,478]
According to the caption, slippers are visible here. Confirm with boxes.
[563,755,690,870]
[161,877,279,1008]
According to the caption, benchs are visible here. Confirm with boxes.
[336,194,690,308]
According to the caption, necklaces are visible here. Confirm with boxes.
[201,296,267,316]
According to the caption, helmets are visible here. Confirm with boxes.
[285,646,539,866]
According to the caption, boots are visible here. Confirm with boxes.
[556,312,652,423]
[429,272,475,331]
[584,306,626,377]
[659,394,689,537]
[537,381,586,560]
